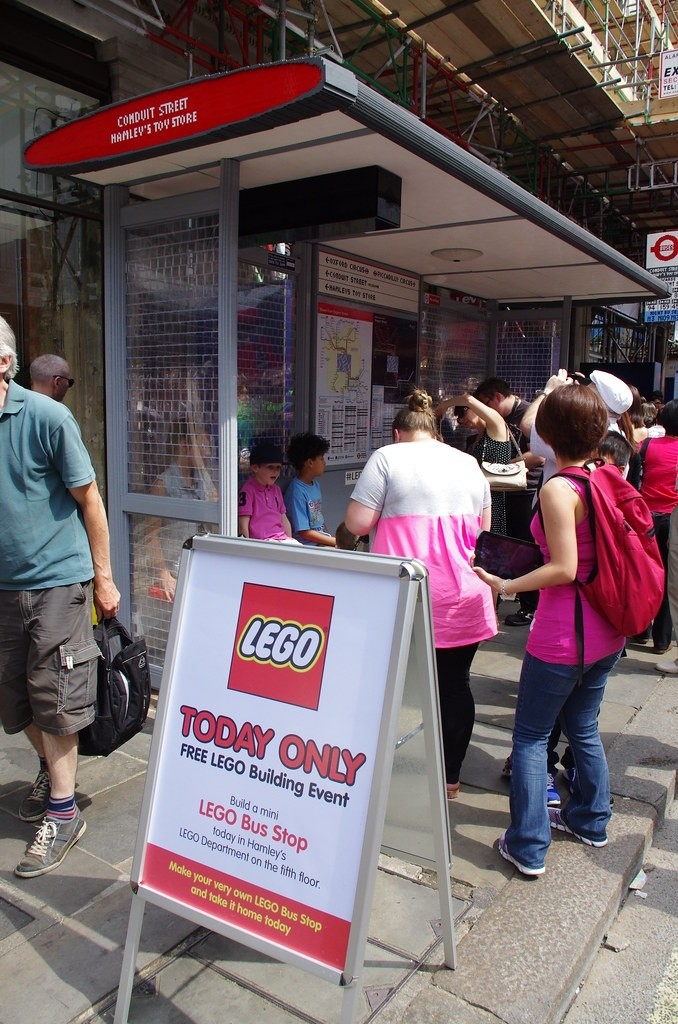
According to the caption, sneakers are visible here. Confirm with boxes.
[14,805,86,877]
[505,609,535,626]
[547,773,562,805]
[19,767,51,821]
[563,767,576,793]
[498,834,546,875]
[548,806,608,847]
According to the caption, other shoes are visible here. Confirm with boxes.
[655,658,678,673]
[653,645,671,653]
[502,757,513,775]
[634,635,649,643]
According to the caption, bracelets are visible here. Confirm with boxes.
[500,579,516,597]
[536,391,547,397]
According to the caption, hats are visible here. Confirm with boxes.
[590,370,634,414]
[250,446,294,465]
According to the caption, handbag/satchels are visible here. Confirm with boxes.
[626,437,652,491]
[480,423,528,492]
[473,531,544,580]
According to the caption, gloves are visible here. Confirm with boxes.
[543,369,573,397]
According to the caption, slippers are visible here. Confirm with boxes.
[447,789,460,799]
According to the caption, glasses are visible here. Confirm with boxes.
[457,407,469,418]
[53,374,75,387]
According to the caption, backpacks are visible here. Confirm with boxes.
[78,614,150,757]
[546,457,665,637]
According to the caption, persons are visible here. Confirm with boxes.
[145,416,220,604]
[422,363,678,672]
[344,387,495,798]
[467,383,627,875]
[30,354,98,629]
[237,445,305,546]
[283,433,337,547]
[0,316,126,875]
